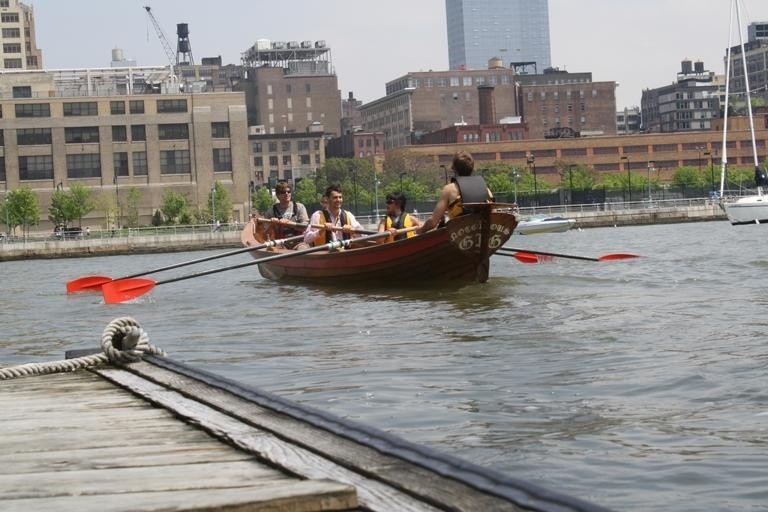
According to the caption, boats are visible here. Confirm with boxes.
[520,216,577,236]
[240,201,516,284]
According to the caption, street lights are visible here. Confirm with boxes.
[569,163,577,201]
[704,152,714,192]
[527,154,539,207]
[648,160,656,200]
[512,169,520,202]
[211,186,217,221]
[398,171,406,190]
[440,164,447,183]
[622,156,631,200]
[372,171,380,223]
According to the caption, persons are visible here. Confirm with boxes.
[374,191,421,246]
[263,179,310,251]
[304,184,366,250]
[413,151,497,234]
[319,194,328,209]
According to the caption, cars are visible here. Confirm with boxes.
[57,226,82,241]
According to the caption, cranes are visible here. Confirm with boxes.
[143,5,196,65]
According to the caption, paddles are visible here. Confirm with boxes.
[67,234,304,293]
[102,226,421,304]
[493,252,538,263]
[500,247,639,260]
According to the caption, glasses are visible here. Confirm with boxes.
[280,190,291,194]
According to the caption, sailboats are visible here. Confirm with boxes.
[721,0,768,224]
